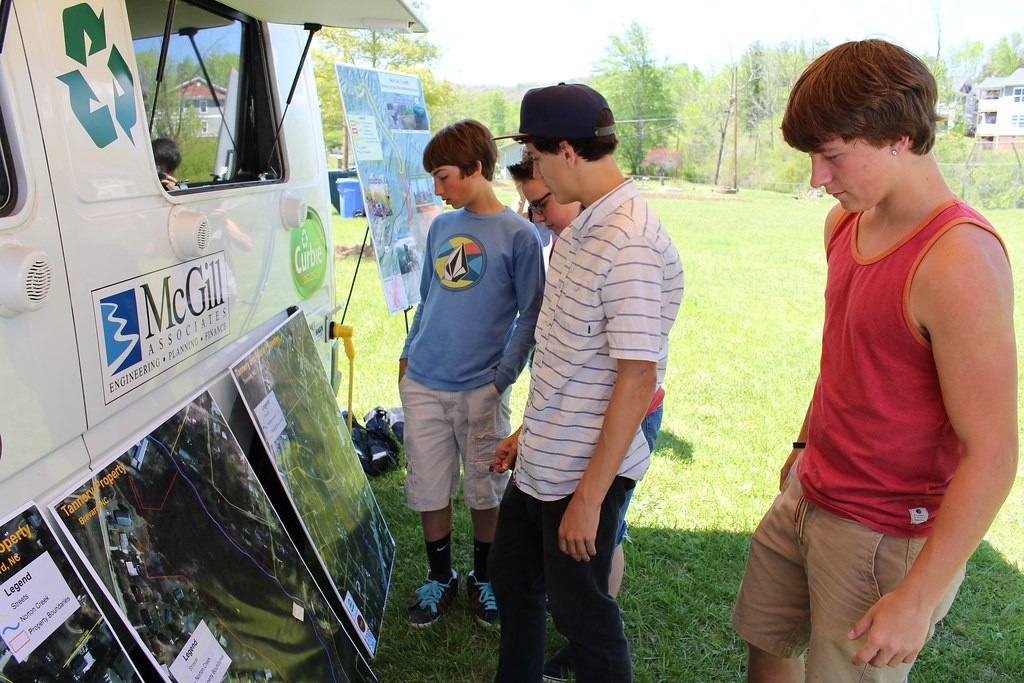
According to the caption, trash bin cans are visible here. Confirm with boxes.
[335,177,366,219]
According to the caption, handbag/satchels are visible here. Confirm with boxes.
[342,405,405,478]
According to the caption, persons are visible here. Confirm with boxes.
[489,81,684,682]
[387,103,396,127]
[728,35,1020,683]
[403,243,411,271]
[151,138,181,189]
[398,117,547,634]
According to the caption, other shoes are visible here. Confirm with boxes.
[542,640,578,683]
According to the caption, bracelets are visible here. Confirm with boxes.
[792,442,807,449]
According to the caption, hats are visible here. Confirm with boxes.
[491,83,615,140]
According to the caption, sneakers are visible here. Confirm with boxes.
[466,569,500,629]
[407,567,458,629]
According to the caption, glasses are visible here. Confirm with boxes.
[528,191,553,216]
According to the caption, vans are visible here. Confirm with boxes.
[1,0,432,522]
